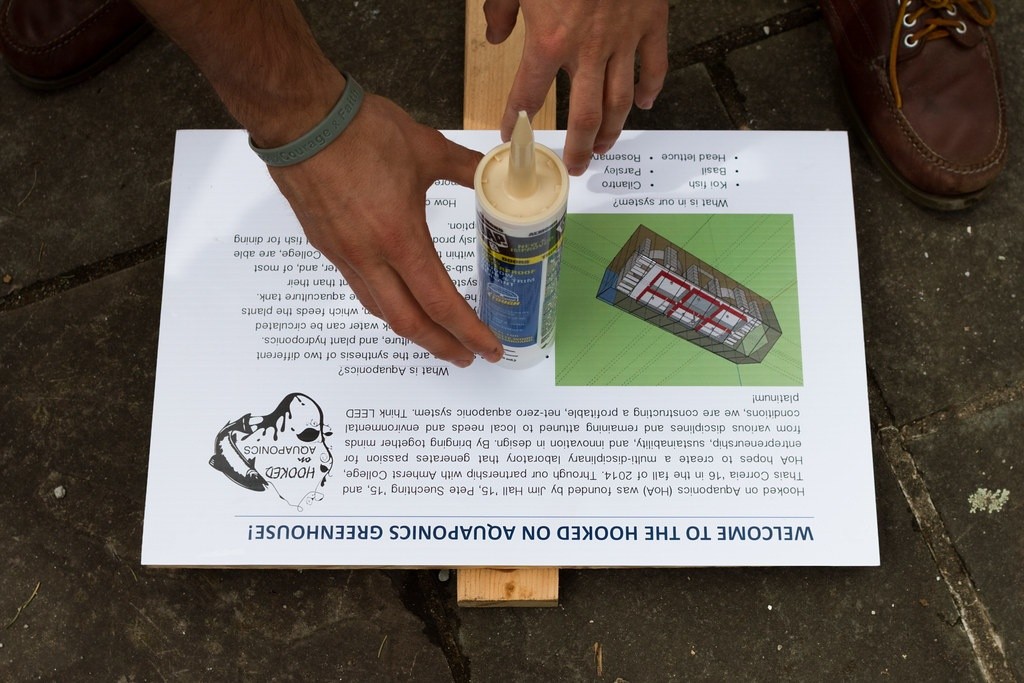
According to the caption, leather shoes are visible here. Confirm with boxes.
[825,0,1008,211]
[0,0,152,95]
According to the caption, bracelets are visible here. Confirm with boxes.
[248,70,365,168]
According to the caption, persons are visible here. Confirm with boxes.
[0,1,1011,365]
